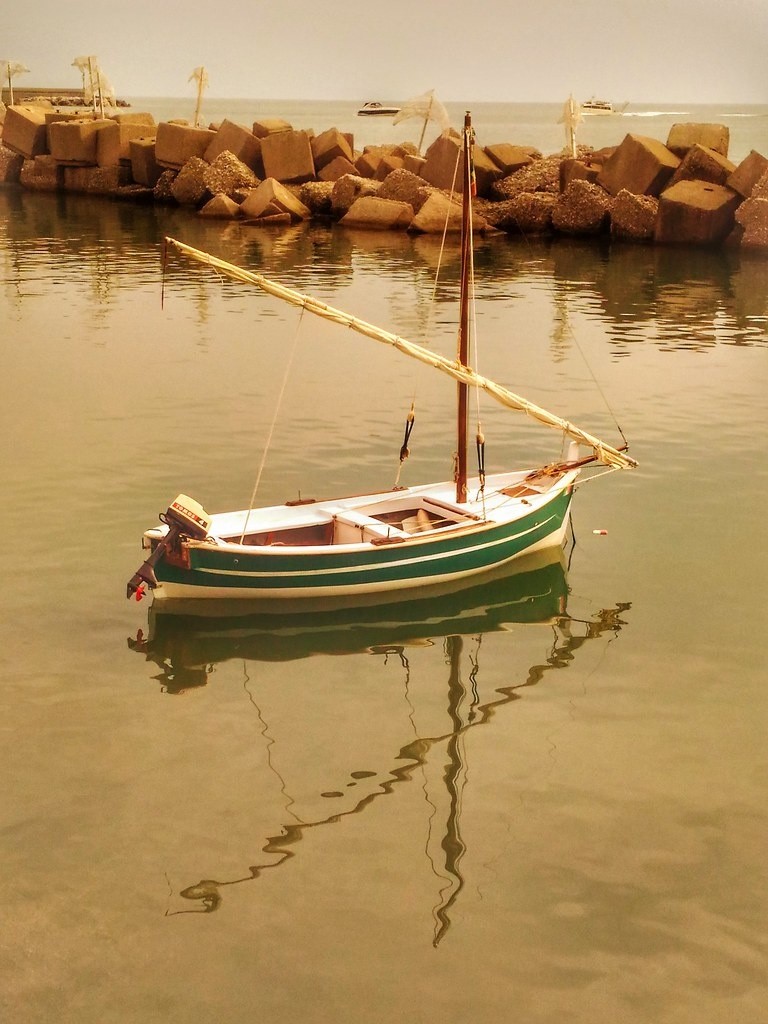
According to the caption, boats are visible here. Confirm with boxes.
[577,97,613,116]
[356,101,402,115]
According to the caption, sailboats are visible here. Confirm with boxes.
[124,108,644,619]
